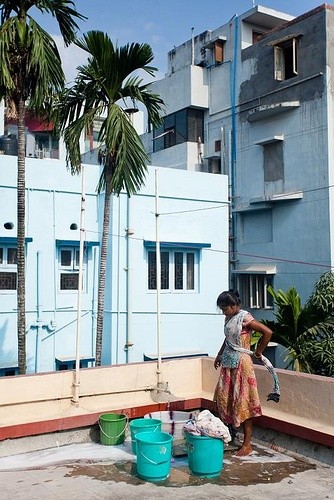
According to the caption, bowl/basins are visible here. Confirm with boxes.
[144,410,196,439]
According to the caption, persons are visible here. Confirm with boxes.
[214,289,273,457]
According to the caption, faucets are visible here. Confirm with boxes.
[164,381,171,395]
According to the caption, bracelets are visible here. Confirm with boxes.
[254,351,262,358]
[217,356,219,358]
[218,353,221,355]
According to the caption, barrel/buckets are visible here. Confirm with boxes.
[98,413,128,446]
[187,435,225,479]
[134,431,174,482]
[129,418,162,455]
